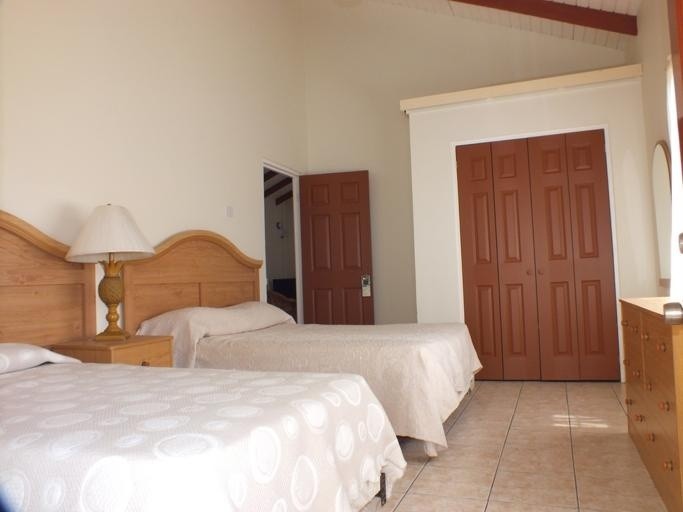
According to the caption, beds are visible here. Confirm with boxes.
[122,230,485,460]
[0,209,407,511]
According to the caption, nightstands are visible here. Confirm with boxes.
[50,334,173,369]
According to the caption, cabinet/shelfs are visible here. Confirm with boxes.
[620,295,683,512]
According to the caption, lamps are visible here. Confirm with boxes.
[64,203,155,341]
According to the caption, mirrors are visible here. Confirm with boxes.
[649,140,672,290]
